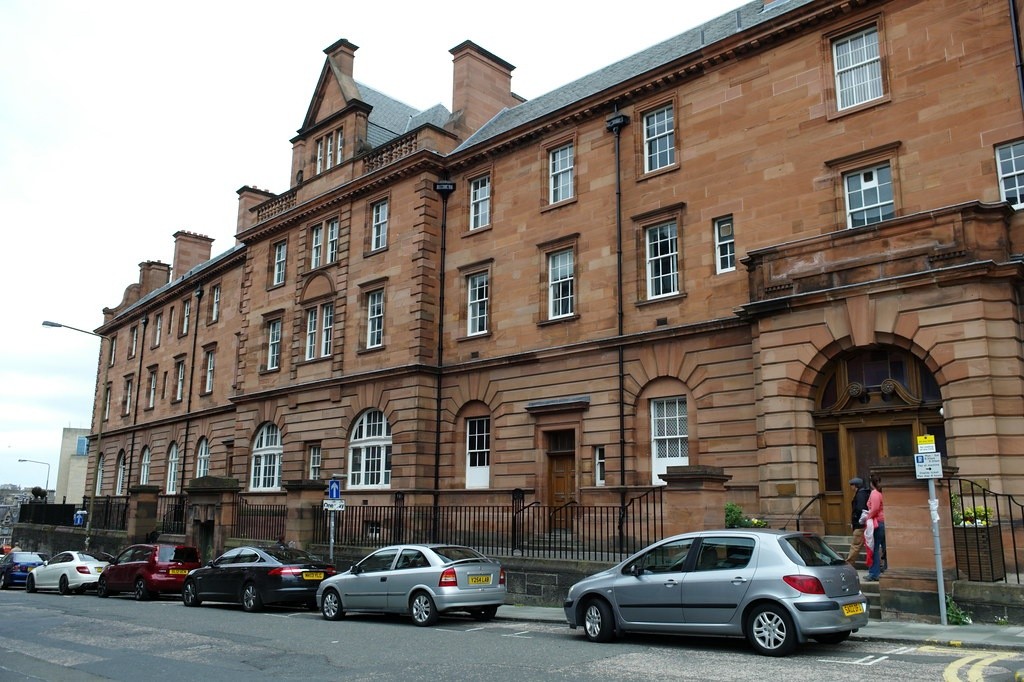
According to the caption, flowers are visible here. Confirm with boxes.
[950,489,993,527]
[724,502,768,528]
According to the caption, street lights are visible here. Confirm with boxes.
[42,320,114,552]
[17,459,51,501]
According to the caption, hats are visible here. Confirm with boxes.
[848,478,864,484]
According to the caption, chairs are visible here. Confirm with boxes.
[701,546,717,566]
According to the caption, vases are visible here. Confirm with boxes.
[952,524,1005,582]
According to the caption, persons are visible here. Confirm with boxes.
[276,537,285,556]
[0,542,22,555]
[845,474,888,582]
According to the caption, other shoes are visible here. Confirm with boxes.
[863,575,879,581]
[880,565,887,572]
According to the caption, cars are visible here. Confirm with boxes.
[0,550,51,590]
[562,528,870,659]
[316,543,510,626]
[96,543,203,600]
[24,550,117,595]
[182,545,338,612]
